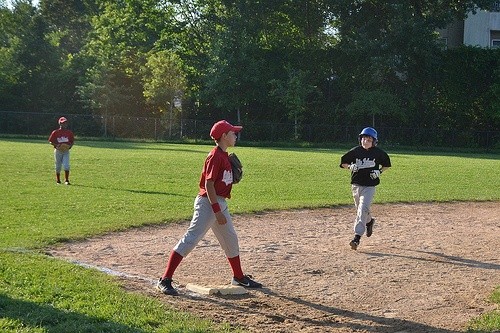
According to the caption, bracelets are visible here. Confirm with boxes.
[211,202,221,213]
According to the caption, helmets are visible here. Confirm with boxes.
[359,127,378,151]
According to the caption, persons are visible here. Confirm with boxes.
[157,120,262,295]
[48,117,74,184]
[338,127,391,250]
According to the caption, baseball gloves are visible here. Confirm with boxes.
[229,153,243,184]
[58,143,70,152]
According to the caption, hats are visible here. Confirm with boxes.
[210,120,243,141]
[58,117,67,125]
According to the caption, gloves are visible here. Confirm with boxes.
[370,169,383,180]
[348,164,358,173]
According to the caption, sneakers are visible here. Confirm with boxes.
[366,217,375,237]
[231,274,263,289]
[350,236,360,250]
[157,277,177,295]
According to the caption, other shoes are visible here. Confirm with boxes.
[57,181,61,184]
[64,180,69,184]
